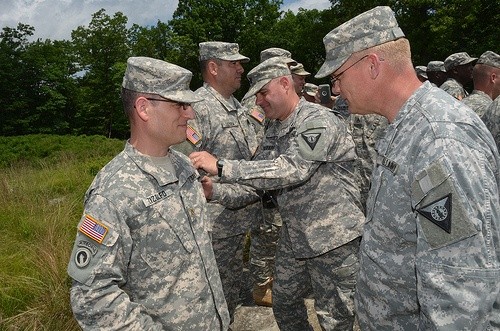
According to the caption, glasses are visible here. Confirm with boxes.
[328,55,385,88]
[134,98,189,110]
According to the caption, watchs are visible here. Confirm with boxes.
[216,157,224,178]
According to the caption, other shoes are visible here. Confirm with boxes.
[252,276,274,307]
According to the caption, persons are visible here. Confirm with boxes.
[166,39,260,331]
[188,4,500,331]
[66,56,233,331]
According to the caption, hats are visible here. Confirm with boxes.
[477,50,500,69]
[313,5,407,78]
[444,51,478,70]
[415,65,429,80]
[239,57,292,102]
[303,83,319,97]
[197,43,251,64]
[427,60,448,72]
[260,47,299,67]
[121,56,205,104]
[290,62,311,75]
